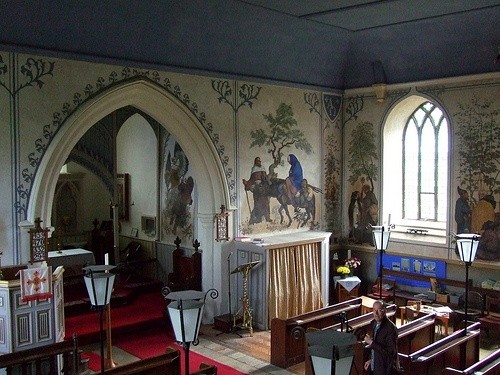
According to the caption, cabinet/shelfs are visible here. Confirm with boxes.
[334,276,361,303]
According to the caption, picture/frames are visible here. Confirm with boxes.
[116,173,128,221]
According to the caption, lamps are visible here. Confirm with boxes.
[84,265,116,372]
[305,330,357,375]
[366,223,395,301]
[447,231,482,335]
[165,291,206,375]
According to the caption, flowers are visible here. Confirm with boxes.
[337,266,350,274]
[343,257,361,270]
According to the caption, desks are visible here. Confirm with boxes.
[407,304,450,336]
[235,229,332,331]
[393,290,458,314]
[46,248,95,288]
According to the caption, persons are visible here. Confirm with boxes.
[363,300,401,375]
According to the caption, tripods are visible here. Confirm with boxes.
[216,252,244,338]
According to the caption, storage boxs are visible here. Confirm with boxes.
[428,289,462,304]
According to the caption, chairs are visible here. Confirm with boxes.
[453,291,500,341]
[116,241,154,283]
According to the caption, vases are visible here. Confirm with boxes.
[349,268,354,276]
[341,274,348,279]
